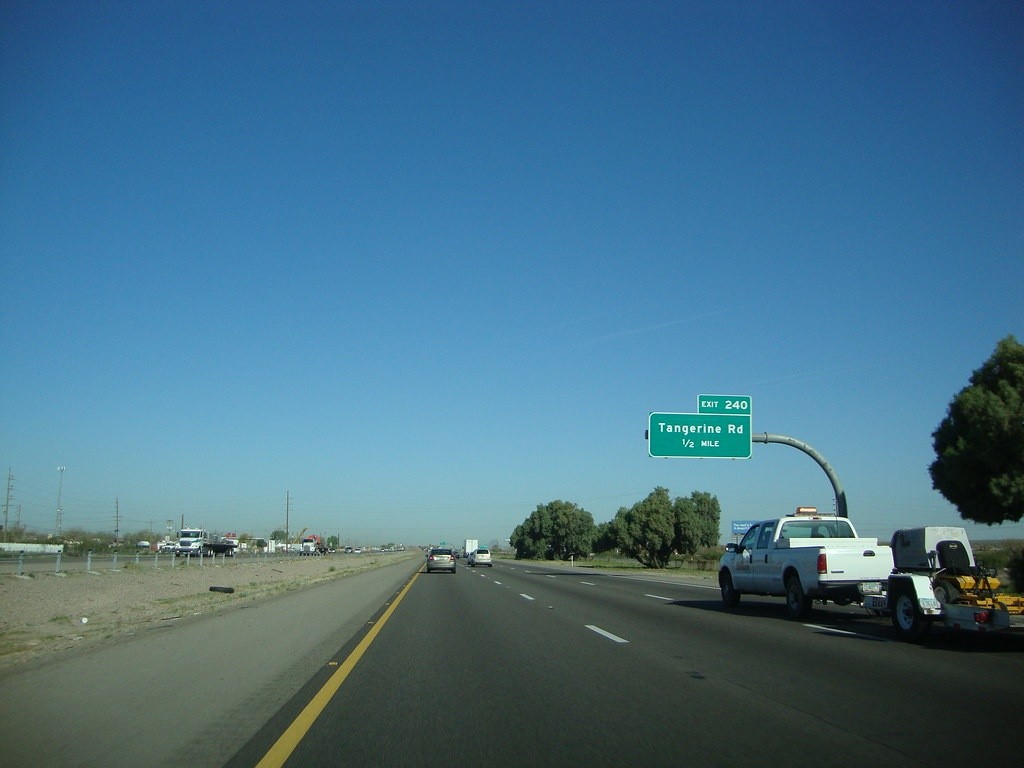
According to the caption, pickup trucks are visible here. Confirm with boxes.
[718,507,895,620]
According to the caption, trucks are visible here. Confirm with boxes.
[462,539,479,558]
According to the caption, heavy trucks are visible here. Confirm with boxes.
[301,538,328,556]
[174,528,239,557]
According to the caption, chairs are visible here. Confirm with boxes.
[810,533,824,538]
[765,531,770,542]
[935,540,981,577]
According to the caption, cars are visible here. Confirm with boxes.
[328,543,405,554]
[424,547,458,574]
[468,548,493,567]
